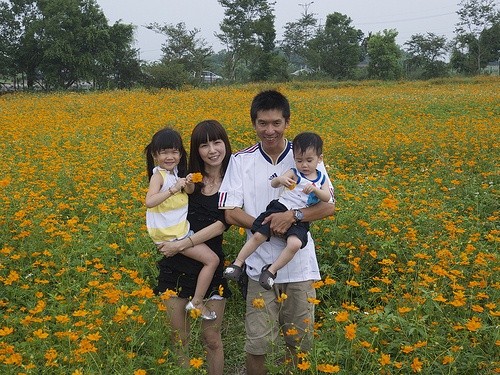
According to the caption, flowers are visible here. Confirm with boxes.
[288,182,296,190]
[189,172,204,184]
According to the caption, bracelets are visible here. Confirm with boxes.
[168,188,174,195]
[187,236,195,247]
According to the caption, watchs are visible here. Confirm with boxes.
[292,209,304,225]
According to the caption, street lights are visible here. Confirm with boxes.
[298,1,314,68]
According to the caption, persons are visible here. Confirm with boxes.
[223,132,331,290]
[155,120,232,375]
[217,89,335,375]
[144,128,220,319]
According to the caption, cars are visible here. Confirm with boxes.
[288,69,311,80]
[192,70,224,83]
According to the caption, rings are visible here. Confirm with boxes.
[278,234,282,236]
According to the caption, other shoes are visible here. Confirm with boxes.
[223,260,246,282]
[259,263,277,289]
[186,302,217,320]
